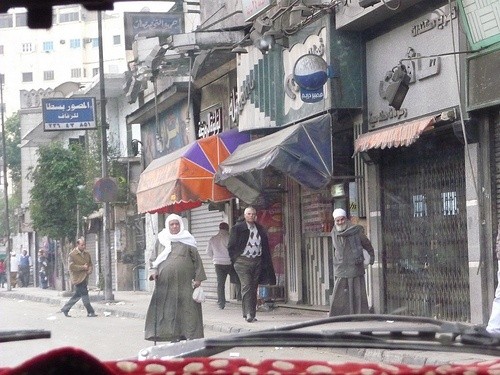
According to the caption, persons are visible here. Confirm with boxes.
[144,214,207,343]
[227,207,276,322]
[0,259,6,288]
[61,238,97,318]
[38,249,49,289]
[328,208,375,317]
[206,221,240,309]
[18,250,32,288]
[10,252,19,289]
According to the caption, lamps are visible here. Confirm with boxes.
[379,48,477,110]
[130,139,142,155]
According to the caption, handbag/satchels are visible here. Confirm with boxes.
[191,279,206,303]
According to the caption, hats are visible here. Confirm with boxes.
[333,209,346,219]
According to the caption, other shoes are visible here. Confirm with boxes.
[247,314,253,322]
[61,309,71,317]
[87,312,97,317]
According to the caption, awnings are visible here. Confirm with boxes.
[136,128,250,214]
[214,113,333,206]
[352,115,436,153]
